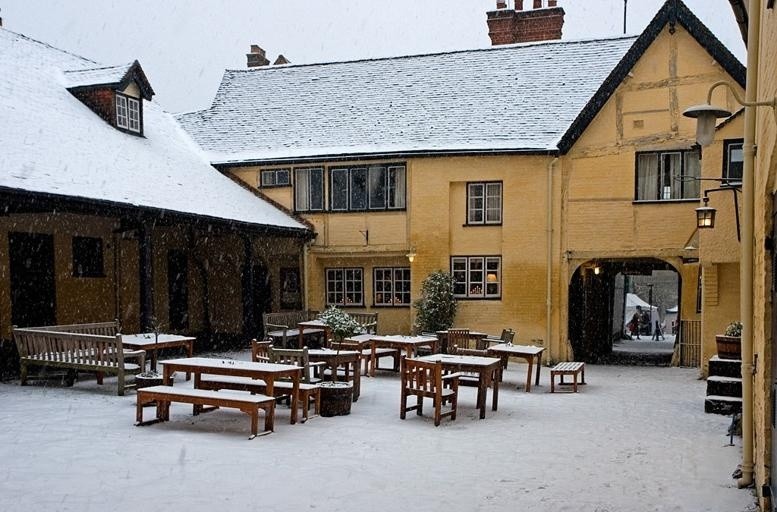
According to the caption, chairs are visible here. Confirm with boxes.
[251,318,545,428]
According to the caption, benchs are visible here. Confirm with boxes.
[549,361,586,393]
[10,317,146,398]
[198,374,320,424]
[134,385,275,441]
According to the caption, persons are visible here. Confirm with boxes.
[631,312,641,340]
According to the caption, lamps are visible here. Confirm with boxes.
[405,245,418,264]
[681,80,776,149]
[694,187,741,244]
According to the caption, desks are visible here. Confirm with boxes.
[158,355,306,433]
[120,332,198,382]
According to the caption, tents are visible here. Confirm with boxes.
[624,293,660,336]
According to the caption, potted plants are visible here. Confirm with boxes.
[409,266,461,358]
[317,303,366,419]
[713,319,741,362]
[131,314,175,408]
